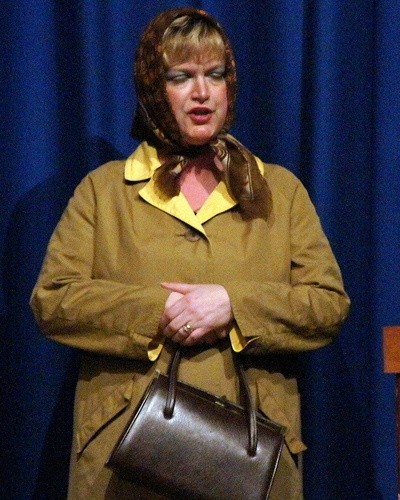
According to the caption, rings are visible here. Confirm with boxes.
[183,324,192,335]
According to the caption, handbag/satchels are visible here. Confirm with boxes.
[104,345,288,500]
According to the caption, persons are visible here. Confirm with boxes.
[30,9,350,500]
